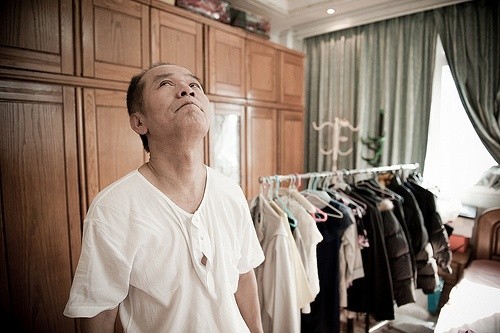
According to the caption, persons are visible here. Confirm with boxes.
[64,63,264,332]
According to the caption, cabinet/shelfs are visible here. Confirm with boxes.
[1,0,310,333]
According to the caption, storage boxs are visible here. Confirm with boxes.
[456,205,478,228]
[452,225,475,238]
[449,234,470,254]
[424,280,453,316]
[436,258,463,281]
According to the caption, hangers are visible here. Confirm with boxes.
[250,164,443,228]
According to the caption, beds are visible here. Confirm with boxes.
[430,204,499,333]
